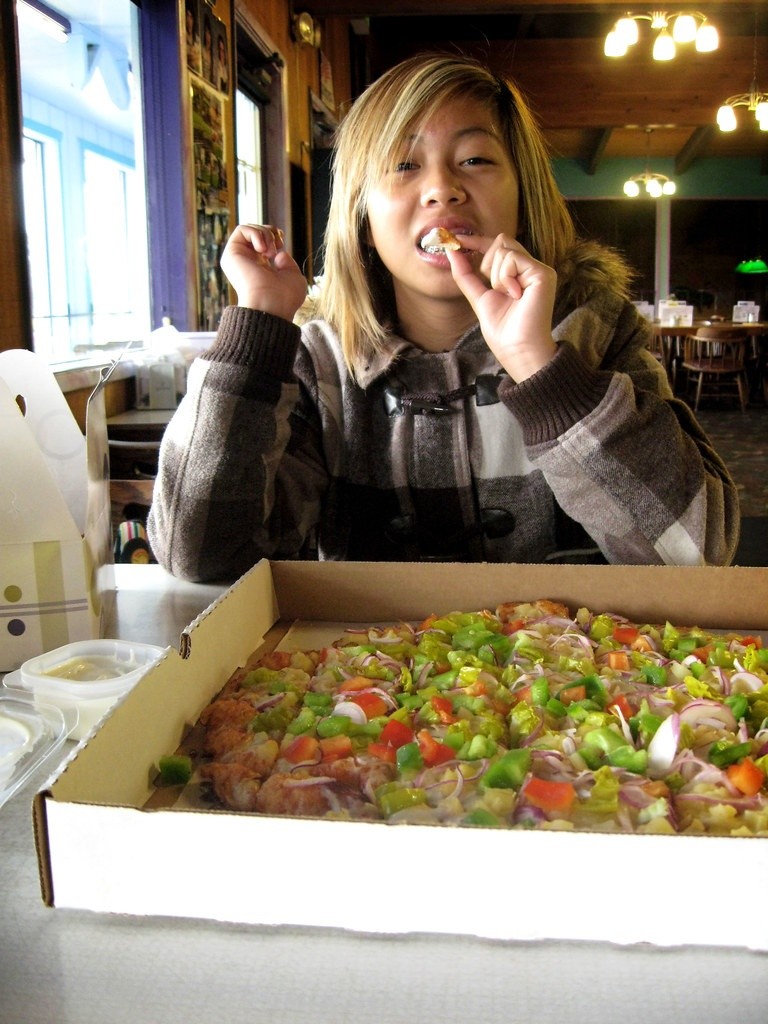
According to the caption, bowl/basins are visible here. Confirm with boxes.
[2,687,78,815]
[21,638,166,741]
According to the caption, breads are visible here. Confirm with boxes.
[420,227,461,250]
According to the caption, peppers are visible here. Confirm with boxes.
[159,755,191,781]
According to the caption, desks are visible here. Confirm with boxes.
[106,410,176,428]
[651,323,768,402]
[1,563,768,1023]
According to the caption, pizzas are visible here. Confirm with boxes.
[199,600,768,838]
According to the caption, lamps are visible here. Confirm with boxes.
[294,13,314,46]
[713,0,768,131]
[623,128,677,197]
[604,0,720,61]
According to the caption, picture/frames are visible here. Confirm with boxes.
[185,0,229,94]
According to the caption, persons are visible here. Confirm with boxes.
[145,52,742,583]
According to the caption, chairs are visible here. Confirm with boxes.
[684,327,751,415]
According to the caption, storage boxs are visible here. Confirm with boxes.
[2,639,165,740]
[0,346,122,670]
[33,558,768,950]
